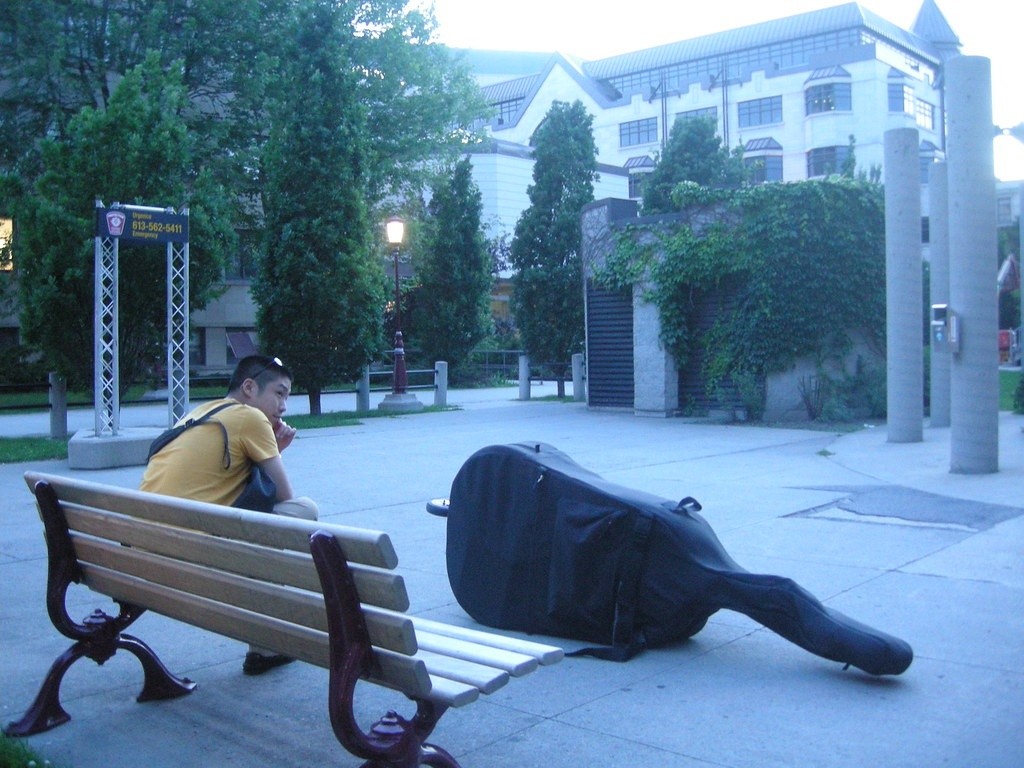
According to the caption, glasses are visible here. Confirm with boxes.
[251,357,283,379]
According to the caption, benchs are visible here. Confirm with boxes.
[4,470,564,768]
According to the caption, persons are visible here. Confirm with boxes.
[141,354,317,674]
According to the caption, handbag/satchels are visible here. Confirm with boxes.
[230,463,276,514]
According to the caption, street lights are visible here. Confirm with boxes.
[383,213,408,393]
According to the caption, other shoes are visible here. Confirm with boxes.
[243,651,295,675]
